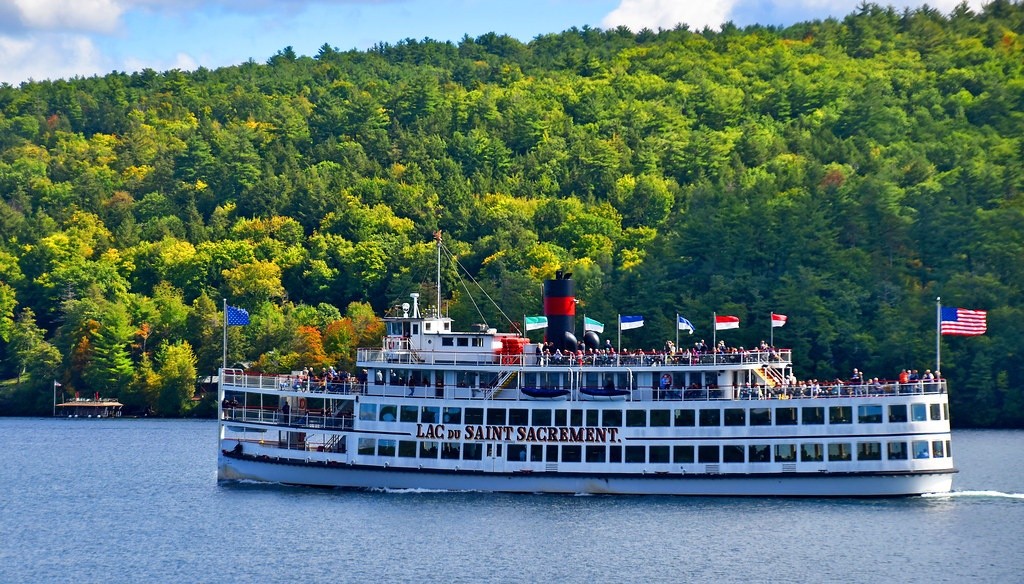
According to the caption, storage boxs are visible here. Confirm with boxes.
[522,344,540,364]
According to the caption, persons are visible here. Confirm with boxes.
[280,365,477,397]
[282,398,535,467]
[542,340,785,367]
[674,412,944,462]
[382,330,410,350]
[536,343,542,367]
[655,369,941,400]
[605,377,614,390]
[222,398,238,420]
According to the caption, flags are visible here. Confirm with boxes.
[620,315,645,331]
[716,315,740,330]
[771,314,788,328]
[55,382,61,386]
[227,304,250,325]
[526,316,548,331]
[585,317,604,333]
[941,304,987,336]
[678,317,696,334]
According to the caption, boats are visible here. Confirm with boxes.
[520,385,571,401]
[52,390,147,418]
[216,229,964,499]
[577,386,631,401]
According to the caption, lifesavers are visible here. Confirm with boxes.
[298,398,306,409]
[502,356,514,365]
[388,340,395,350]
